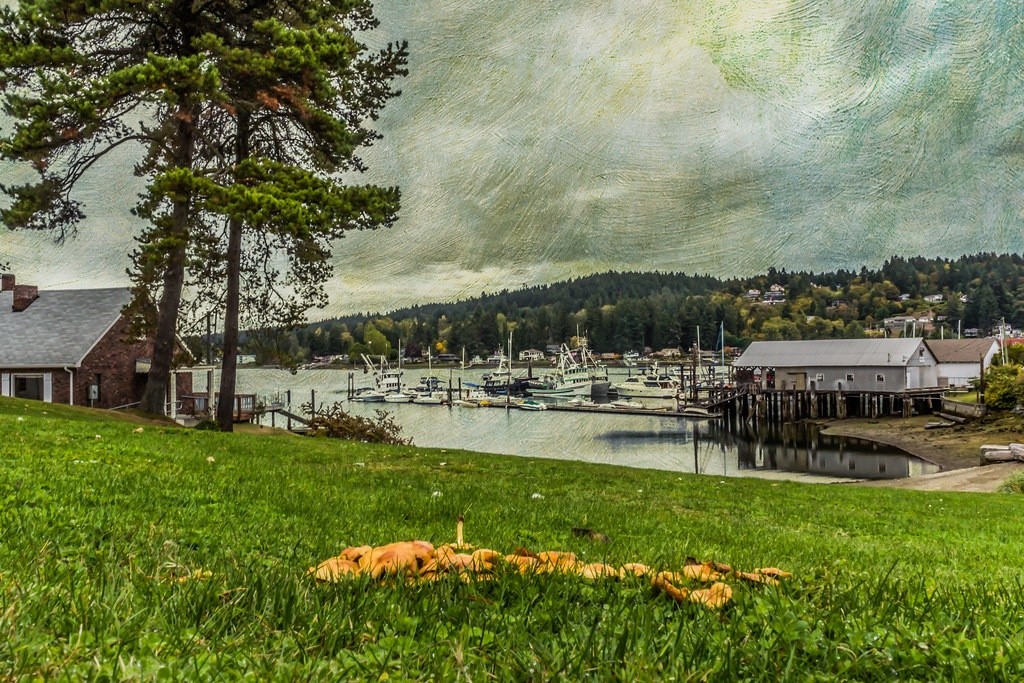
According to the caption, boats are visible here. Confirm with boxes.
[349,387,384,402]
[413,396,441,404]
[462,349,516,397]
[403,373,451,395]
[384,394,411,403]
[526,324,611,397]
[612,362,682,398]
[361,353,401,394]
